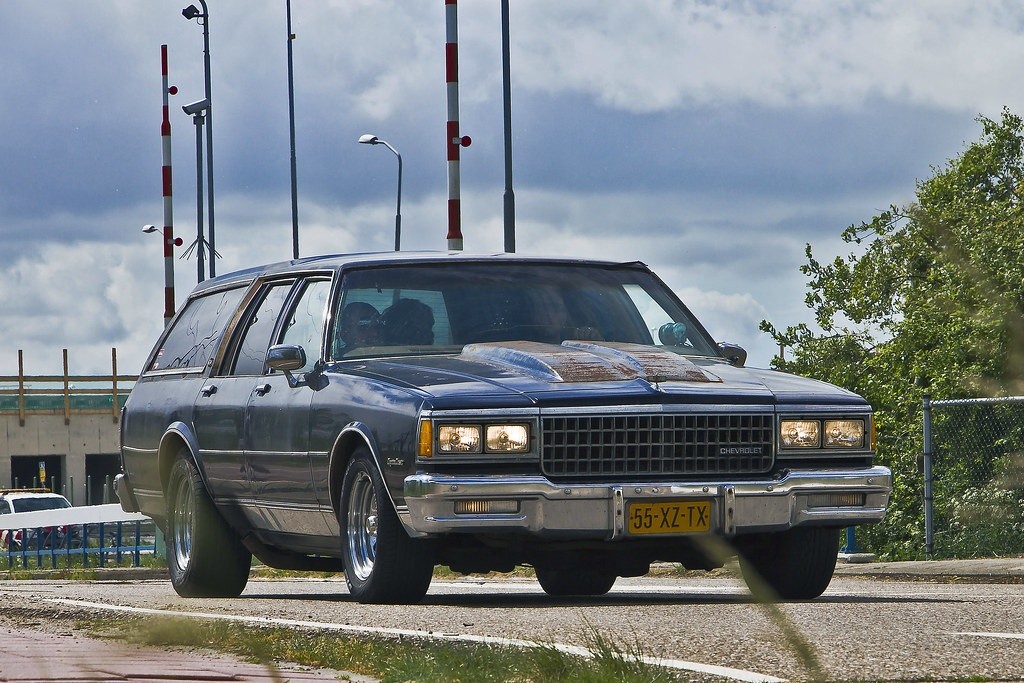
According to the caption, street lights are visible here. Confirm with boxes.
[358,134,402,305]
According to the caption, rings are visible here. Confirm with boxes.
[576,328,579,330]
[587,327,591,330]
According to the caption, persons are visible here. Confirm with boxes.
[340,302,386,349]
[529,283,605,344]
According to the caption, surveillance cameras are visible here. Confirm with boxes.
[182,98,210,115]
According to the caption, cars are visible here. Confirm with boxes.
[0,487,89,550]
[112,248,893,604]
